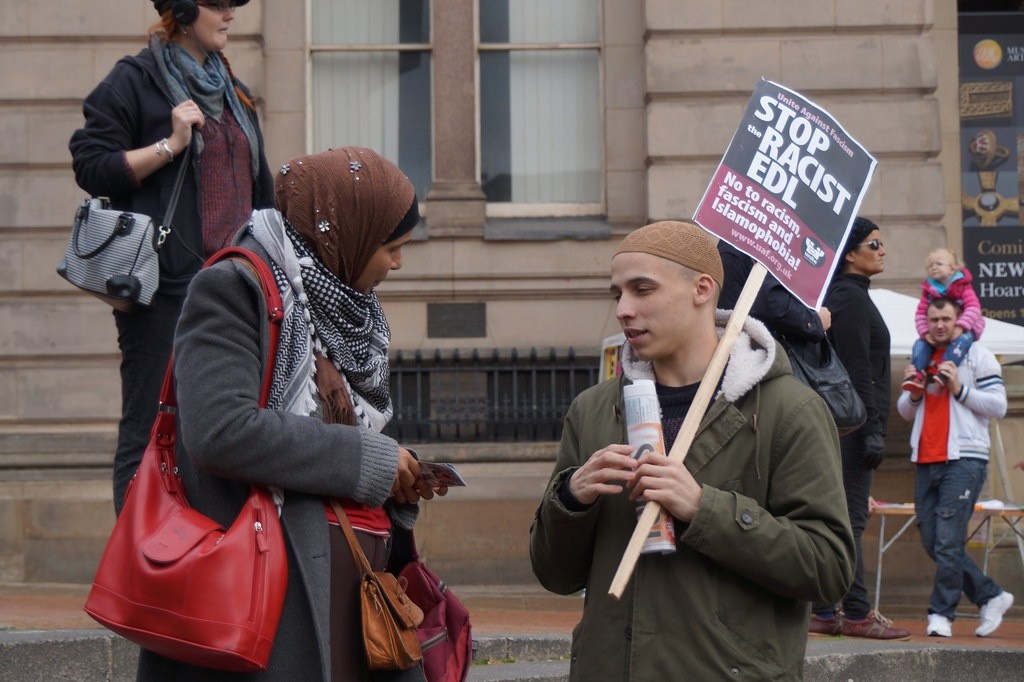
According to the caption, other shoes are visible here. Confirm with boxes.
[902,375,924,393]
[933,373,947,387]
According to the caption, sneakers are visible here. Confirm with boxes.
[927,614,952,636]
[809,611,845,637]
[842,610,912,640]
[975,591,1015,636]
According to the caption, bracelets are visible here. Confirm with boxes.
[153,137,175,161]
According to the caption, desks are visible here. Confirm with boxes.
[867,508,1024,612]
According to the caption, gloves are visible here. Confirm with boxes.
[862,432,885,471]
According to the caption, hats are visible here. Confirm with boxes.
[841,216,879,255]
[152,0,249,10]
[611,221,724,289]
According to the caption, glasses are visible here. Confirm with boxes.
[856,240,883,250]
[194,0,235,13]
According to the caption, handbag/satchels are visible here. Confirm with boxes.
[57,196,159,312]
[360,573,424,669]
[786,339,868,436]
[399,559,472,682]
[82,247,288,672]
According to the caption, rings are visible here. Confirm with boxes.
[409,502,418,504]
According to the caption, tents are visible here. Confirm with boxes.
[863,285,1024,564]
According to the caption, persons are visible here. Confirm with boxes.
[807,214,907,640]
[529,217,857,681]
[135,146,448,682]
[896,302,1016,640]
[901,246,986,395]
[65,0,274,523]
[712,236,832,385]
